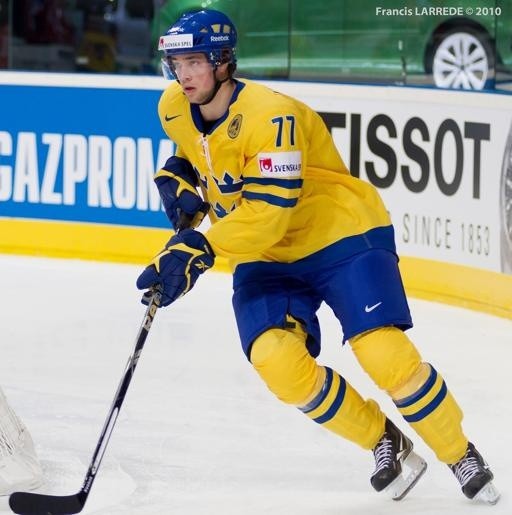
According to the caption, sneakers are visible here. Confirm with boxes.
[446,441,494,500]
[369,416,414,492]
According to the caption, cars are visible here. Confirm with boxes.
[149,0,512,92]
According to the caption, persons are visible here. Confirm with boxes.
[134,6,494,500]
[72,13,117,74]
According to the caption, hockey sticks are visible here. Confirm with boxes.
[10,207,196,515]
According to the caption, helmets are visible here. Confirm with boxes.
[157,8,238,81]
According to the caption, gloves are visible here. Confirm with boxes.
[136,226,215,307]
[153,155,202,233]
